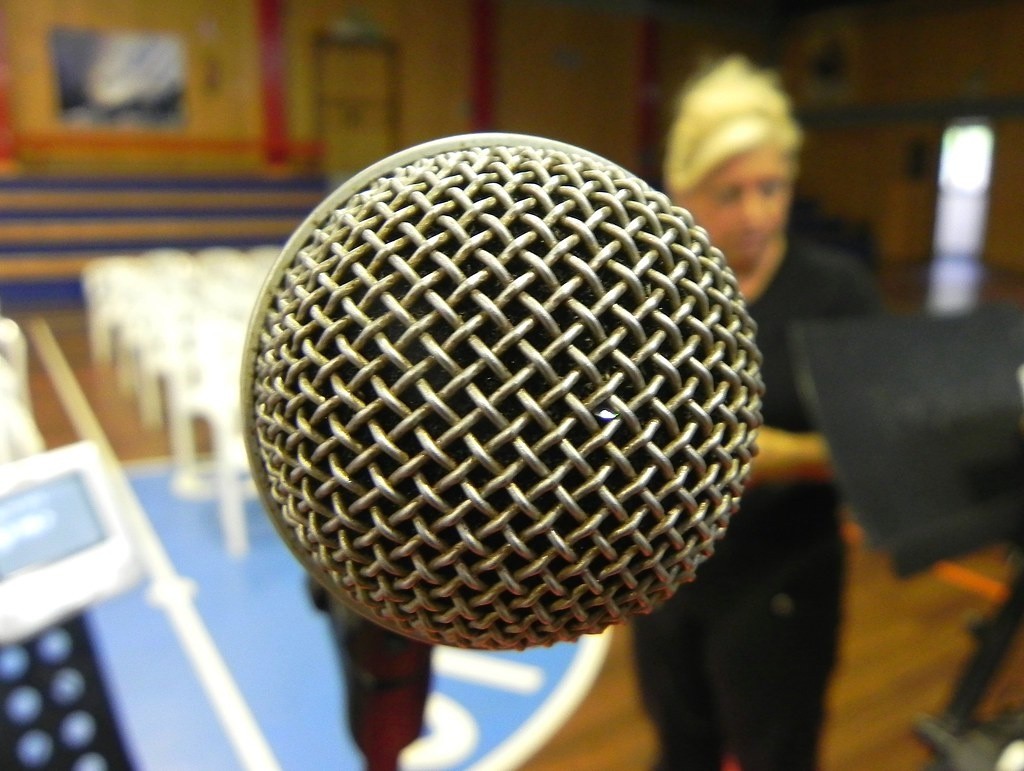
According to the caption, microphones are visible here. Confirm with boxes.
[235,130,767,648]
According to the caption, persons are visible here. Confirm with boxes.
[628,52,865,769]
